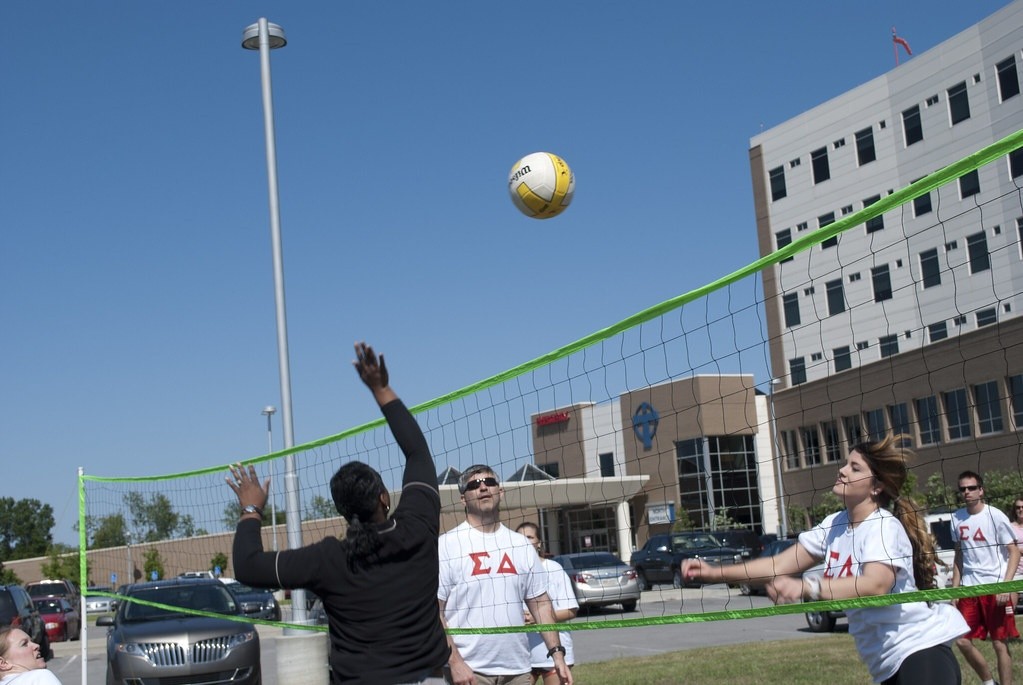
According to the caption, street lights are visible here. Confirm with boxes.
[261,404,285,605]
[242,16,330,685]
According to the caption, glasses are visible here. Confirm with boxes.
[1015,506,1023,509]
[959,486,982,492]
[462,478,499,494]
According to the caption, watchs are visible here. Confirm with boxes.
[806,571,822,601]
[240,504,262,515]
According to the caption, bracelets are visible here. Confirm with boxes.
[546,646,566,658]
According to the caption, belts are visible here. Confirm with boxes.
[425,666,443,677]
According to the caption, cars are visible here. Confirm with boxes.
[739,534,799,598]
[25,572,291,642]
[550,552,640,616]
[97,578,262,685]
[630,532,752,591]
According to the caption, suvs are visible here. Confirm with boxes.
[801,513,962,632]
[0,584,50,660]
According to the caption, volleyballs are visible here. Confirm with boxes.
[507,151,577,220]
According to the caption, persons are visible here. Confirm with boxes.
[0,626,62,685]
[223,342,453,685]
[436,464,580,685]
[950,470,1023,685]
[680,439,971,685]
